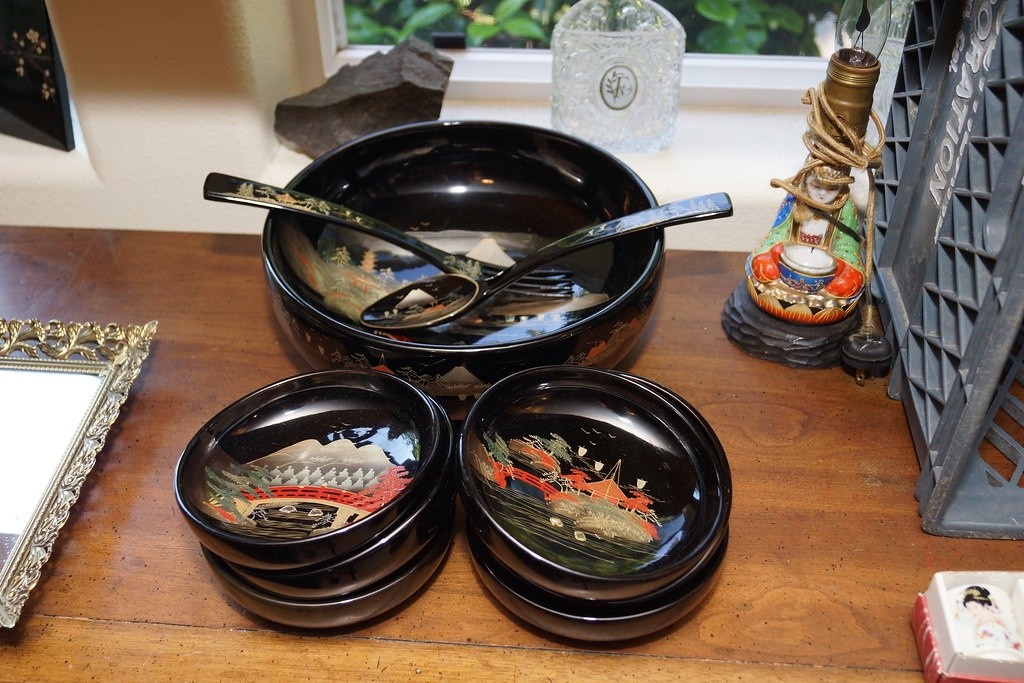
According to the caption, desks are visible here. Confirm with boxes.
[0,223,1024,683]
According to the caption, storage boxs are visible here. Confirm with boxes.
[861,0,1024,540]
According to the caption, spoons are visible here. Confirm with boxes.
[361,193,734,331]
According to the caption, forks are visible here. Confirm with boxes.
[204,173,574,300]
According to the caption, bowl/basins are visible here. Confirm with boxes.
[174,368,455,627]
[454,365,732,642]
[260,120,666,418]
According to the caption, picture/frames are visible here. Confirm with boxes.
[0,318,159,629]
